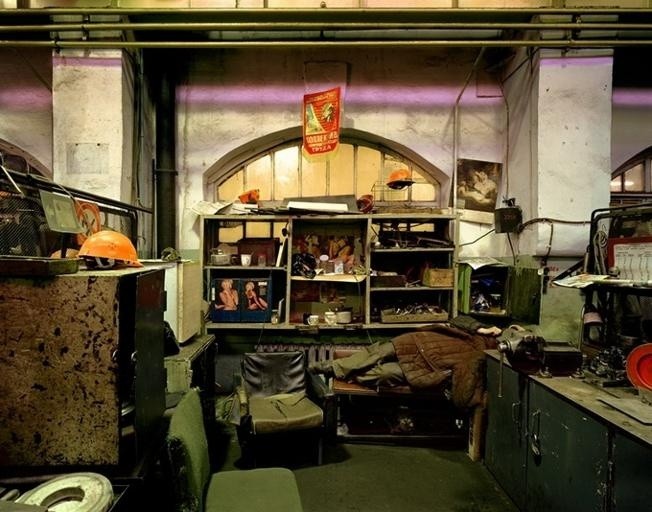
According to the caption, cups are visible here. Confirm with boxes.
[307,314,319,326]
[240,254,252,266]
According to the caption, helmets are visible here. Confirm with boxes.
[77,230,145,267]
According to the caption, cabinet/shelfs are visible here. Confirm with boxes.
[198,212,460,337]
[457,258,542,326]
[483,357,652,512]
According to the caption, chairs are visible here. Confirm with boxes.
[234,350,324,466]
[168,389,302,511]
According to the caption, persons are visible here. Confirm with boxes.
[310,316,531,388]
[244,282,268,310]
[457,159,503,204]
[219,279,239,310]
